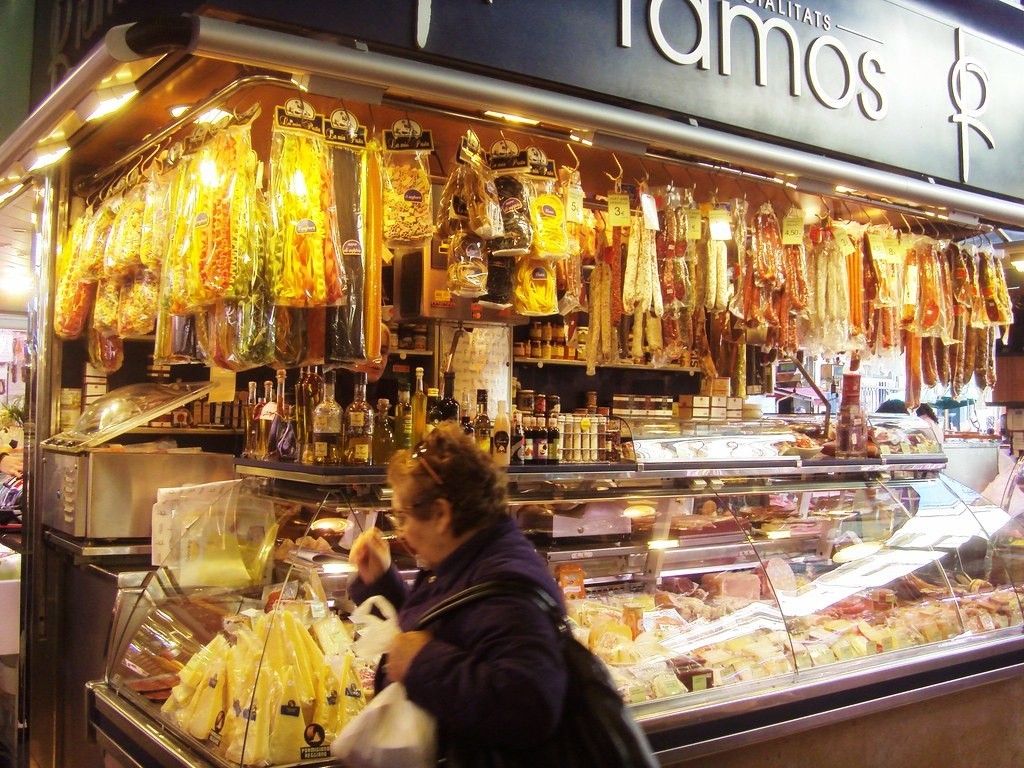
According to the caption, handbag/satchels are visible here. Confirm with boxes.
[330,681,437,768]
[414,574,665,768]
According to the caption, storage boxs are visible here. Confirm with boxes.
[80,362,108,416]
[679,395,743,419]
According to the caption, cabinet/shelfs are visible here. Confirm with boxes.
[51,242,703,433]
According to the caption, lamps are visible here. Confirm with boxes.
[16,138,71,173]
[74,83,140,123]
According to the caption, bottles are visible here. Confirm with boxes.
[173,378,248,429]
[244,365,622,467]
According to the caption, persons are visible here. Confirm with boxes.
[346,421,570,767]
[917,404,944,444]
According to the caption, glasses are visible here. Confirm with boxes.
[411,440,445,486]
